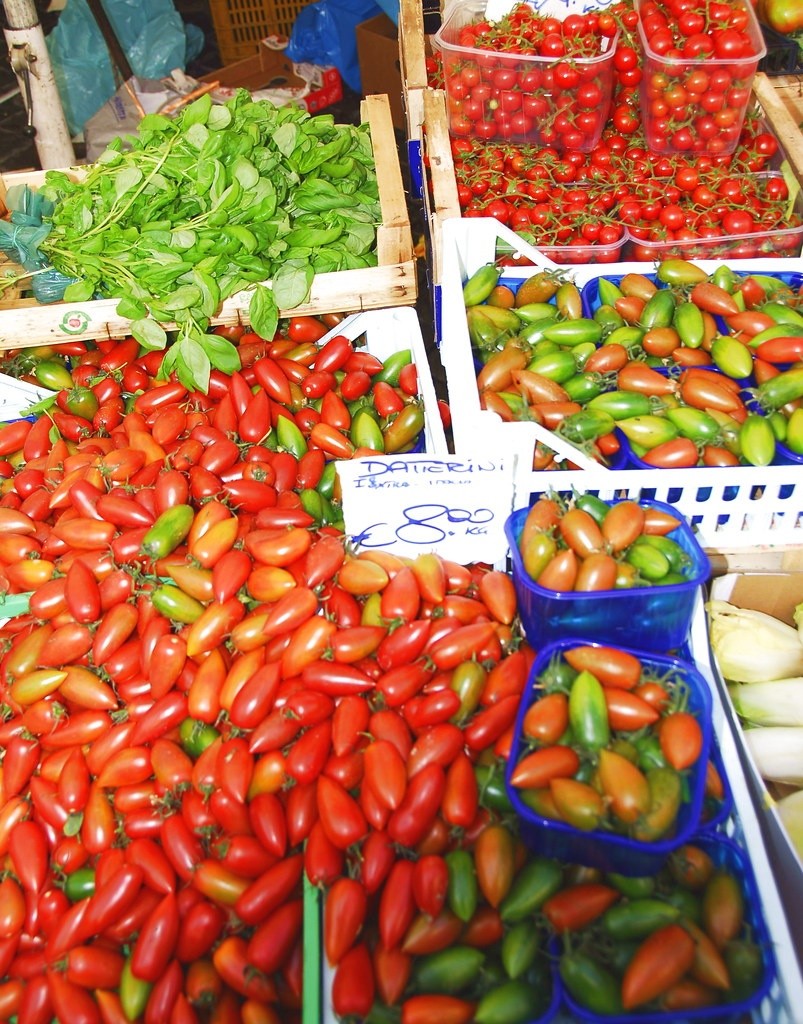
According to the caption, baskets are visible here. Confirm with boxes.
[437,217,802,555]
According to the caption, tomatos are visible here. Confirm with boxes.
[0,309,764,1024]
[420,0,803,475]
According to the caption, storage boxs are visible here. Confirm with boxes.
[503,497,710,657]
[354,14,404,130]
[707,570,803,970]
[315,305,450,456]
[504,636,713,879]
[632,0,768,158]
[197,35,344,113]
[553,830,776,1023]
[577,272,731,370]
[432,0,622,154]
[495,224,627,268]
[0,94,419,350]
[611,372,757,470]
[437,216,802,550]
[627,106,803,262]
[319,584,803,1024]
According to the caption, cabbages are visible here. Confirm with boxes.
[700,596,803,866]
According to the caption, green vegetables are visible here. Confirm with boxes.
[0,87,384,397]
